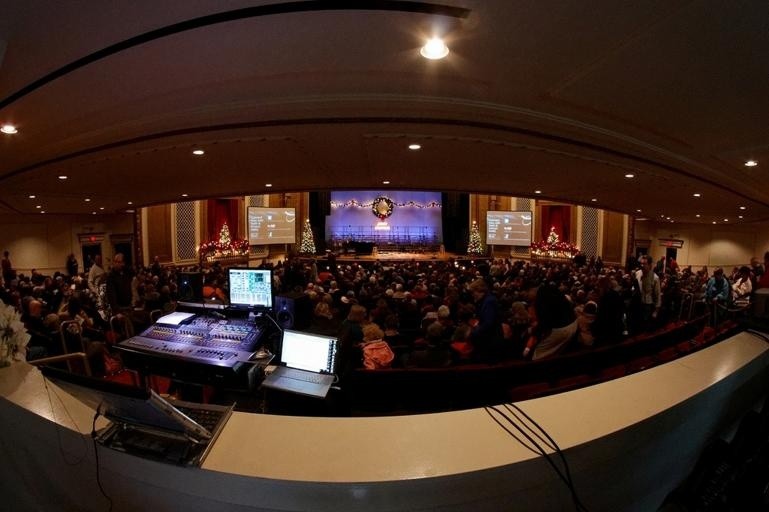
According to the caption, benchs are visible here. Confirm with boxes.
[25,292,715,414]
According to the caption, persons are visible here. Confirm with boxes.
[1,250,376,376]
[303,251,768,377]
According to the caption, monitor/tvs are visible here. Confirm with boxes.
[226,267,275,310]
[176,272,203,303]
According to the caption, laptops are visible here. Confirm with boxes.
[261,328,341,398]
